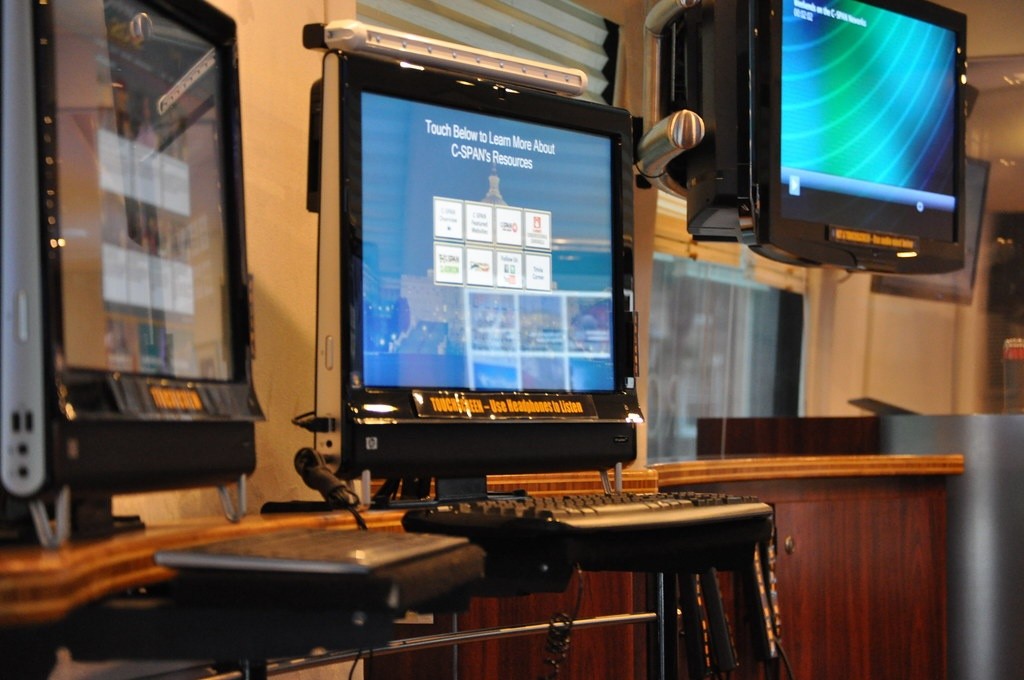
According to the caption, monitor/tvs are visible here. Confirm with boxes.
[870,156,990,304]
[660,0,968,274]
[309,48,638,510]
[0,1,267,549]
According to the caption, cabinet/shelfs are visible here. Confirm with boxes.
[365,574,651,680]
[675,475,946,680]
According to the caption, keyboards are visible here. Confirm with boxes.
[152,529,485,613]
[400,492,772,537]
